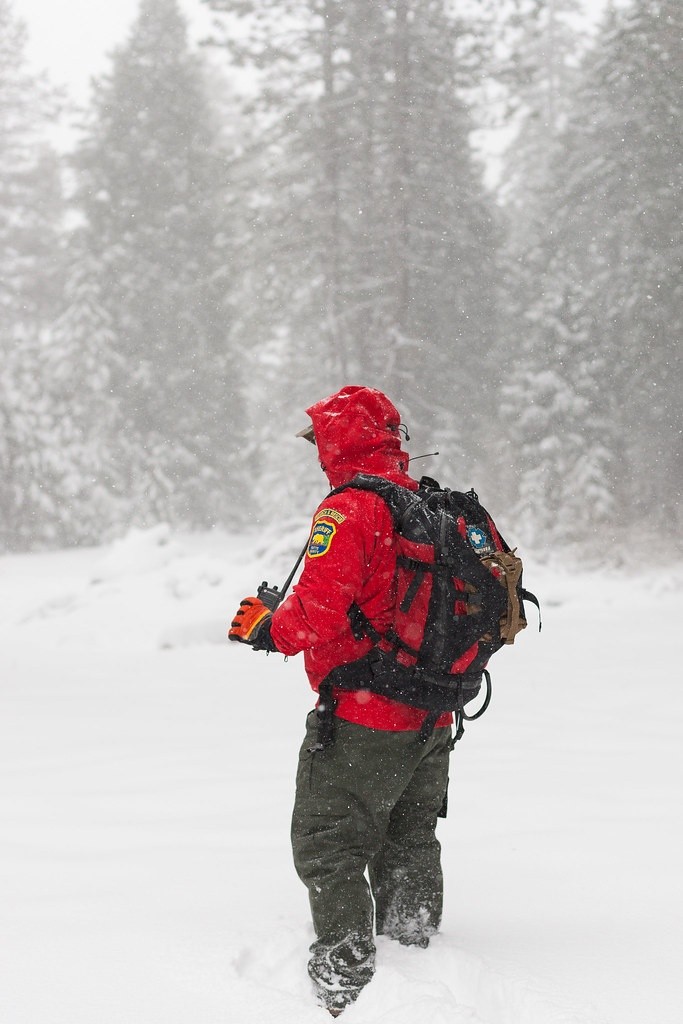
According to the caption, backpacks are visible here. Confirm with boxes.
[322,476,544,751]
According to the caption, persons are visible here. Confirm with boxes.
[228,385,452,1018]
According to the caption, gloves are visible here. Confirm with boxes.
[228,597,279,656]
